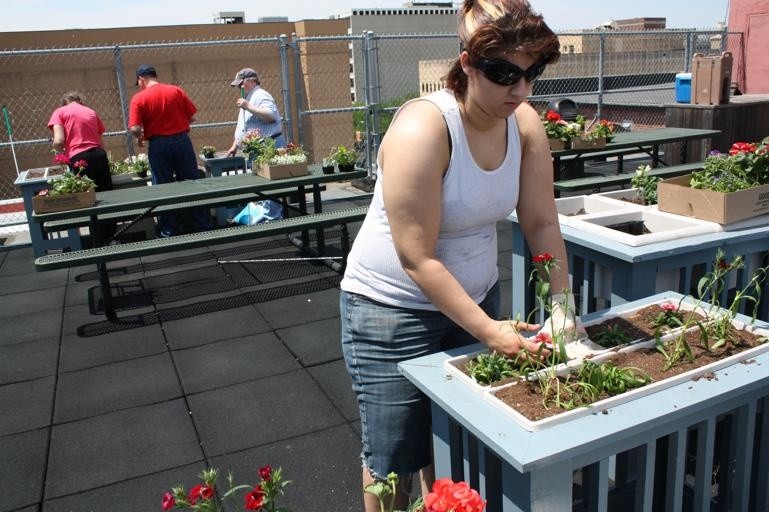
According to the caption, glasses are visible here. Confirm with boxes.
[463,54,548,85]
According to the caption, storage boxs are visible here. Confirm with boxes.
[675,72,692,102]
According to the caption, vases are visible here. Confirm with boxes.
[252,160,308,180]
[32,188,97,214]
[136,170,148,177]
[548,138,565,150]
[444,297,769,430]
[565,136,606,149]
[657,169,769,225]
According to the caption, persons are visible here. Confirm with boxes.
[49,91,116,244]
[128,63,214,240]
[225,67,288,223]
[337,1,579,512]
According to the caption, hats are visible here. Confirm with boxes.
[134,65,155,86]
[230,68,259,85]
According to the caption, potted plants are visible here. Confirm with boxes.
[198,143,217,158]
[322,156,336,174]
[328,144,360,172]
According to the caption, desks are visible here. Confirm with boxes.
[32,162,368,313]
[541,127,722,199]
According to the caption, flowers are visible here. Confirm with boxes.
[458,250,769,410]
[161,464,292,512]
[124,153,150,172]
[362,472,488,512]
[236,126,304,164]
[691,137,769,191]
[592,119,616,138]
[37,153,98,204]
[630,164,660,199]
[542,109,568,138]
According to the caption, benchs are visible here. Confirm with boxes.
[552,145,653,162]
[583,150,665,169]
[553,162,708,194]
[43,183,326,282]
[34,205,372,339]
